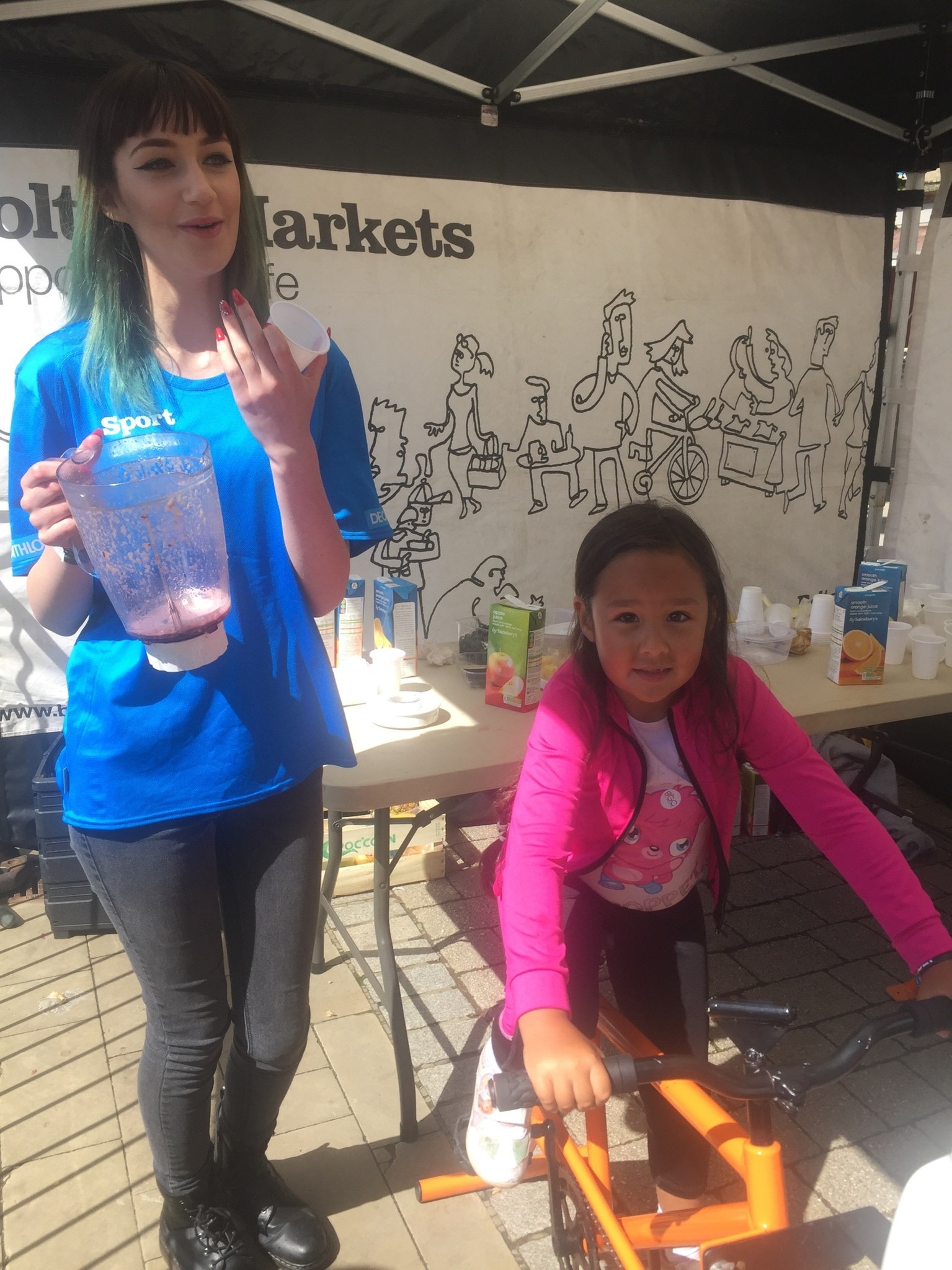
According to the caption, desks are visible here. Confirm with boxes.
[306,616,952,1146]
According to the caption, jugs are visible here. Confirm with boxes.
[55,431,232,673]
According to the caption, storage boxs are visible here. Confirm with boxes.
[30,730,119,942]
[307,800,448,898]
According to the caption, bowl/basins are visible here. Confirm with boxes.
[726,620,798,666]
[456,651,487,688]
[539,610,581,686]
[456,614,490,665]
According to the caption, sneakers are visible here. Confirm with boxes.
[464,1034,532,1188]
[642,1210,737,1270]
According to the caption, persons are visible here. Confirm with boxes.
[7,62,400,1269]
[466,505,952,1270]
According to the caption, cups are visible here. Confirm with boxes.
[807,594,835,645]
[369,648,406,697]
[266,302,330,372]
[884,581,952,680]
[735,586,794,637]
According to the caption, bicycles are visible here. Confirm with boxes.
[414,940,952,1270]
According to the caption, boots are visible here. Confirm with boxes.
[219,1158,342,1270]
[159,1182,273,1270]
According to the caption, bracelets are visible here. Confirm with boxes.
[52,547,91,566]
[915,951,952,984]
